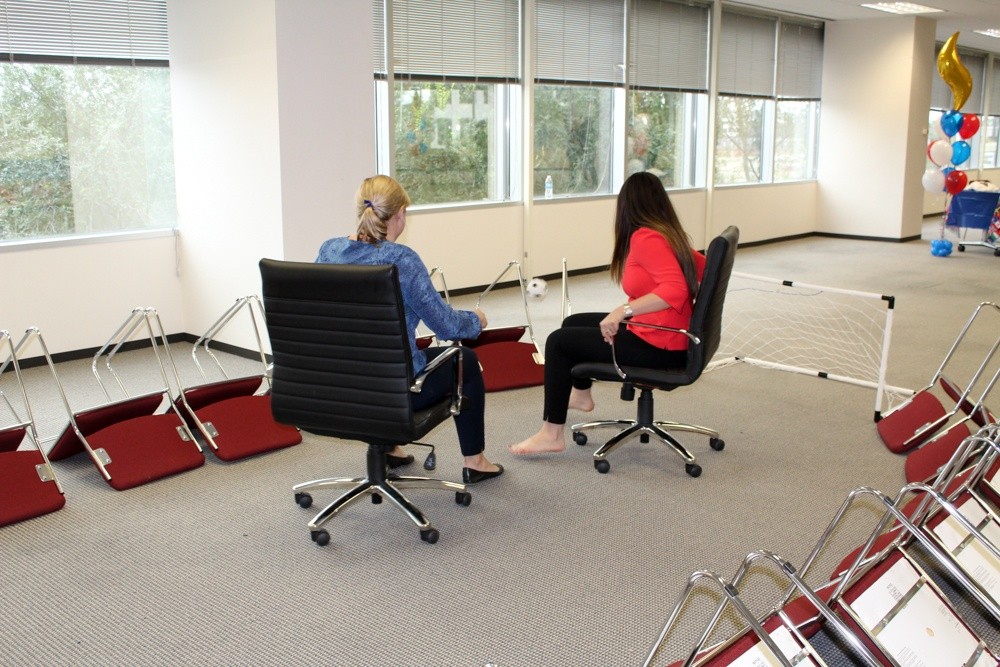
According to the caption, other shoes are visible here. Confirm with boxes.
[384,454,414,469]
[463,463,504,483]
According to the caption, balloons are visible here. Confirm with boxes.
[923,30,981,257]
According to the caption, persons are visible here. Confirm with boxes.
[315,174,505,483]
[508,171,705,456]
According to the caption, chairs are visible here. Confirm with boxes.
[0,226,999,666]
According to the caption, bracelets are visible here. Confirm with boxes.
[624,303,633,318]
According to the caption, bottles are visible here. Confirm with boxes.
[544,176,553,199]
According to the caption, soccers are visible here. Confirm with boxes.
[526,278,549,302]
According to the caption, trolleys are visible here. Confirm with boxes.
[951,179,1000,257]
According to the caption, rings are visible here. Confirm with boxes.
[607,330,612,334]
